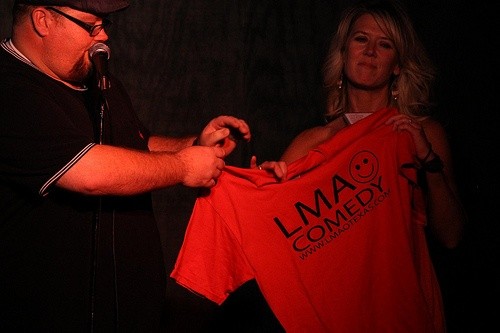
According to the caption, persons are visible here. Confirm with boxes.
[249,0,464,249]
[0,0,253,333]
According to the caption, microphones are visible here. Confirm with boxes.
[88,42,111,96]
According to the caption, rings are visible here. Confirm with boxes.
[259,165,262,170]
[409,120,412,124]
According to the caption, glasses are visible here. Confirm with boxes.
[45,6,112,36]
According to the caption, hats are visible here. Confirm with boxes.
[17,0,130,15]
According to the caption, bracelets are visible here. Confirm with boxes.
[415,141,445,174]
[192,137,199,146]
